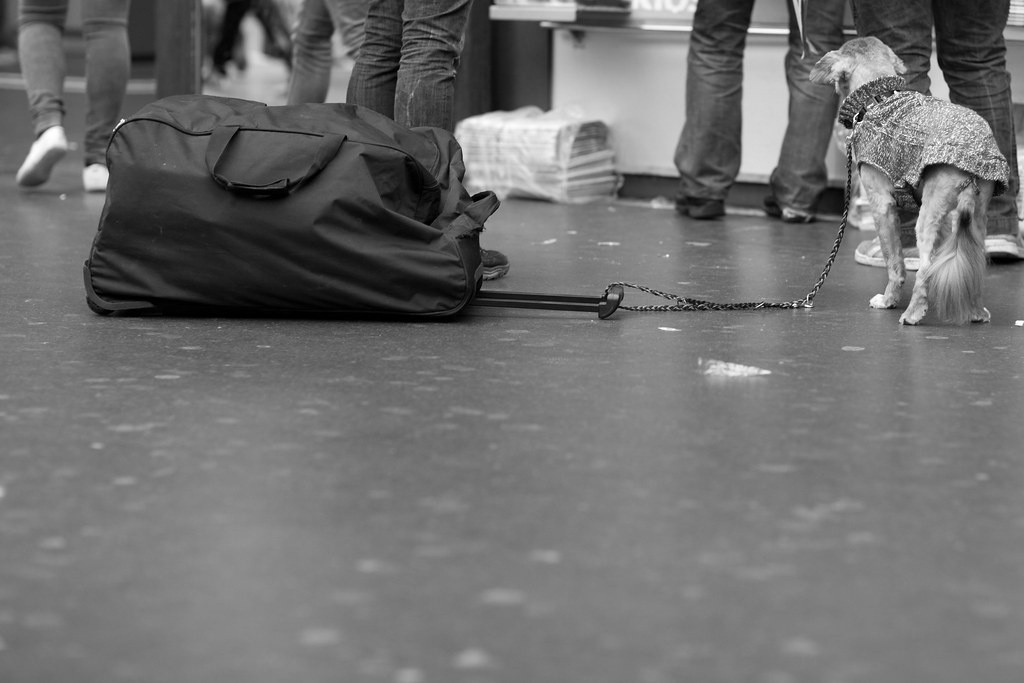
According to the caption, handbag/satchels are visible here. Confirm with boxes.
[81,92,500,324]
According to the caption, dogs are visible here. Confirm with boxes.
[808,35,994,325]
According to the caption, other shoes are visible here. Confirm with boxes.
[81,161,110,192]
[672,191,724,218]
[17,125,68,185]
[480,242,511,279]
[764,194,814,222]
[847,201,877,232]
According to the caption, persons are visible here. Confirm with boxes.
[15,0,132,195]
[672,0,846,226]
[282,0,510,283]
[850,0,1024,272]
[205,0,292,88]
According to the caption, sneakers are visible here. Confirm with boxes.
[984,221,1024,261]
[853,233,921,270]
